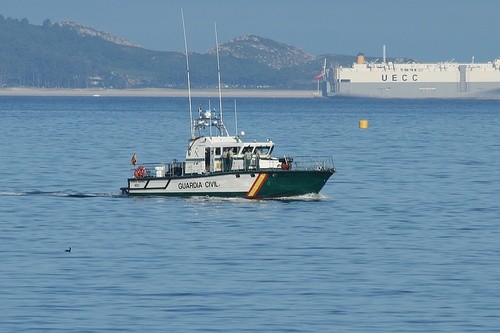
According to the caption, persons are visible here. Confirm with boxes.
[244,148,252,171]
[222,147,234,172]
[255,147,261,172]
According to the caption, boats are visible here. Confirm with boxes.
[120,9,337,200]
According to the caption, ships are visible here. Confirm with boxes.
[313,58,500,99]
